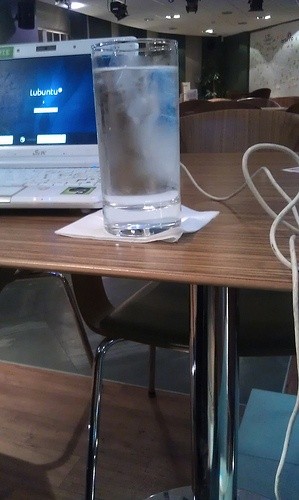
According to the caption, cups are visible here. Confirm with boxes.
[91,38,182,239]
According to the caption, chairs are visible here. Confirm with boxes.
[180,88,299,116]
[72,108,299,500]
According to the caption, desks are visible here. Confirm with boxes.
[0,153,299,500]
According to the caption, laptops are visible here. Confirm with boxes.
[0,37,139,209]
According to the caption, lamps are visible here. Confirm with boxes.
[248,0,264,11]
[110,0,130,22]
[185,0,198,13]
